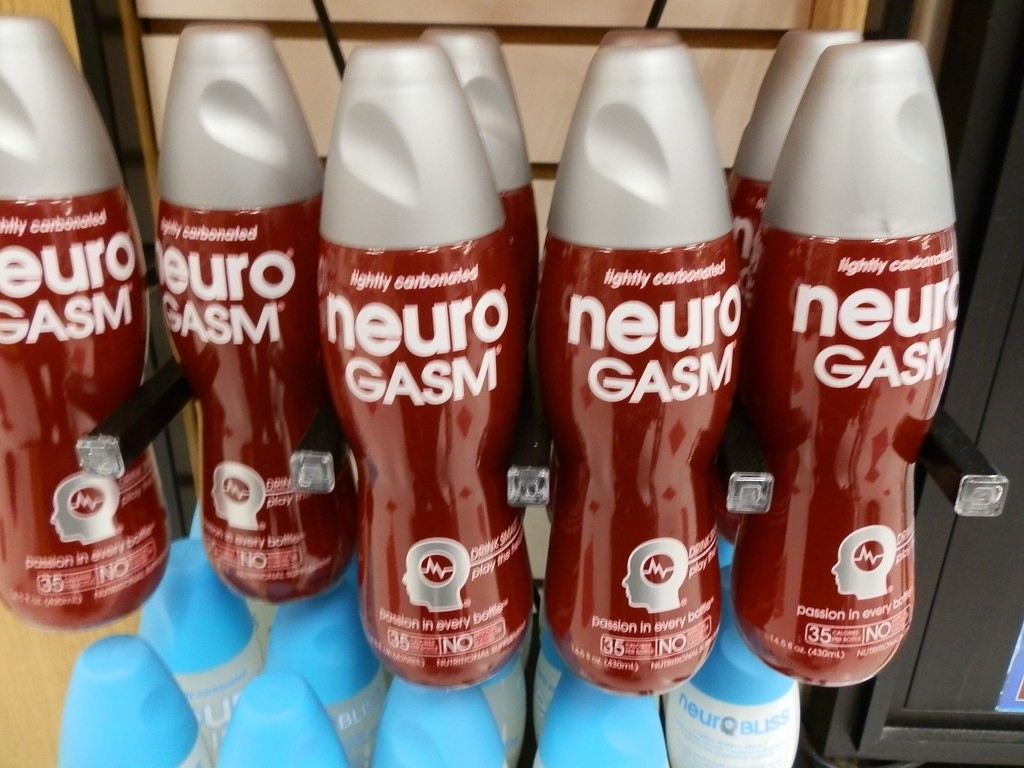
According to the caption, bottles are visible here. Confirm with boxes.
[55,504,802,766]
[0,16,170,629]
[714,24,868,548]
[420,23,539,489]
[153,21,355,604]
[730,38,960,687]
[316,42,535,686]
[541,33,745,697]
